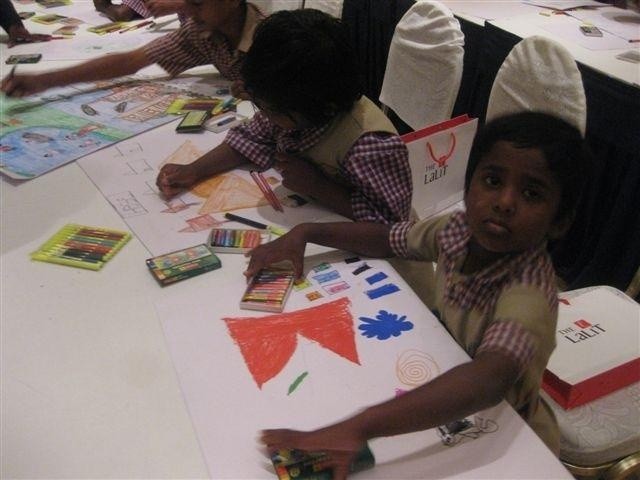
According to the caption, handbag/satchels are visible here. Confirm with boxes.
[399,114,478,222]
[543,287,640,410]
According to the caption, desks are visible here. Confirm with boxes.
[414,0,640,99]
[1,2,574,477]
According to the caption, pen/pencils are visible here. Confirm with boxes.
[4,60,19,100]
[242,267,294,314]
[163,172,170,201]
[249,169,279,212]
[214,97,235,114]
[257,170,284,213]
[217,117,235,126]
[224,212,285,236]
[130,20,153,31]
[206,226,263,259]
[29,221,133,270]
[222,98,243,113]
[119,21,148,33]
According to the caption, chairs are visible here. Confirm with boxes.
[480,32,590,152]
[251,2,346,21]
[373,3,469,139]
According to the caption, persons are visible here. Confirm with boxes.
[241,108,588,472]
[157,7,413,226]
[92,1,178,23]
[0,0,262,101]
[0,1,52,50]
